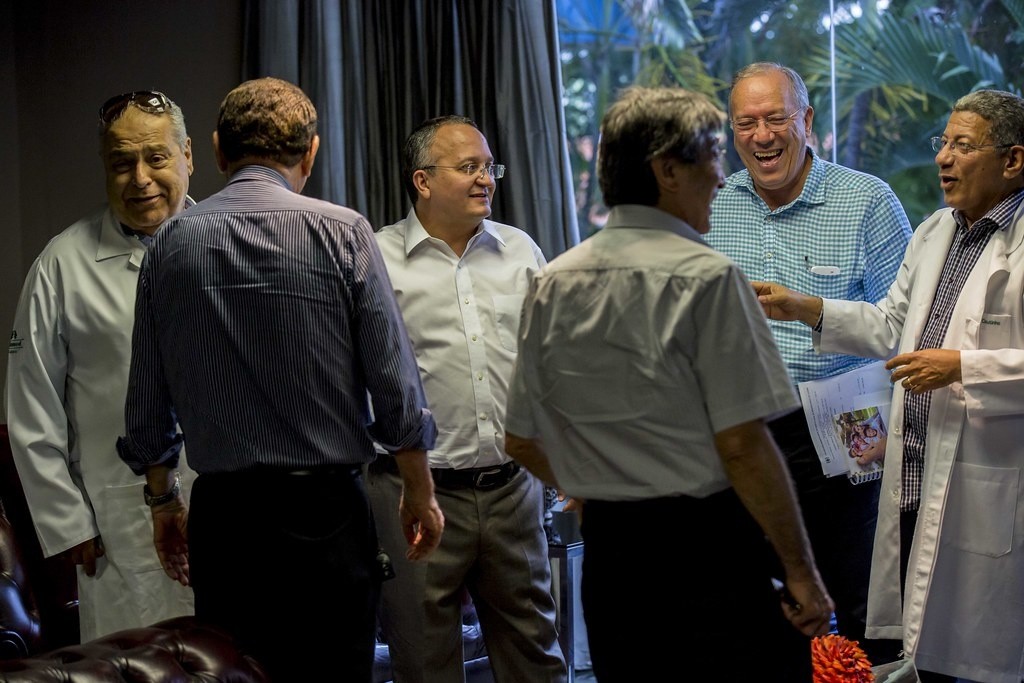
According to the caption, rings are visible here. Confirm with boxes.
[907,378,917,387]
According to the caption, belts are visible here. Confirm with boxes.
[199,466,372,492]
[376,456,522,489]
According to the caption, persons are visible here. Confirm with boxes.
[505,87,835,683]
[116,76,445,683]
[848,411,888,458]
[3,90,198,649]
[699,62,914,667]
[362,114,578,683]
[749,89,1024,683]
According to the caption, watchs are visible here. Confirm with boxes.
[143,472,182,507]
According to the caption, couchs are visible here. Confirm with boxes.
[0,498,271,683]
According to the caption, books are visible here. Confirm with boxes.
[831,406,888,486]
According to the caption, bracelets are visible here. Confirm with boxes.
[813,310,823,334]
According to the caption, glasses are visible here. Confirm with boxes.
[423,163,507,179]
[98,91,173,123]
[730,107,804,134]
[931,136,1012,157]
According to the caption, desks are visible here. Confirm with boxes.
[546,541,587,683]
